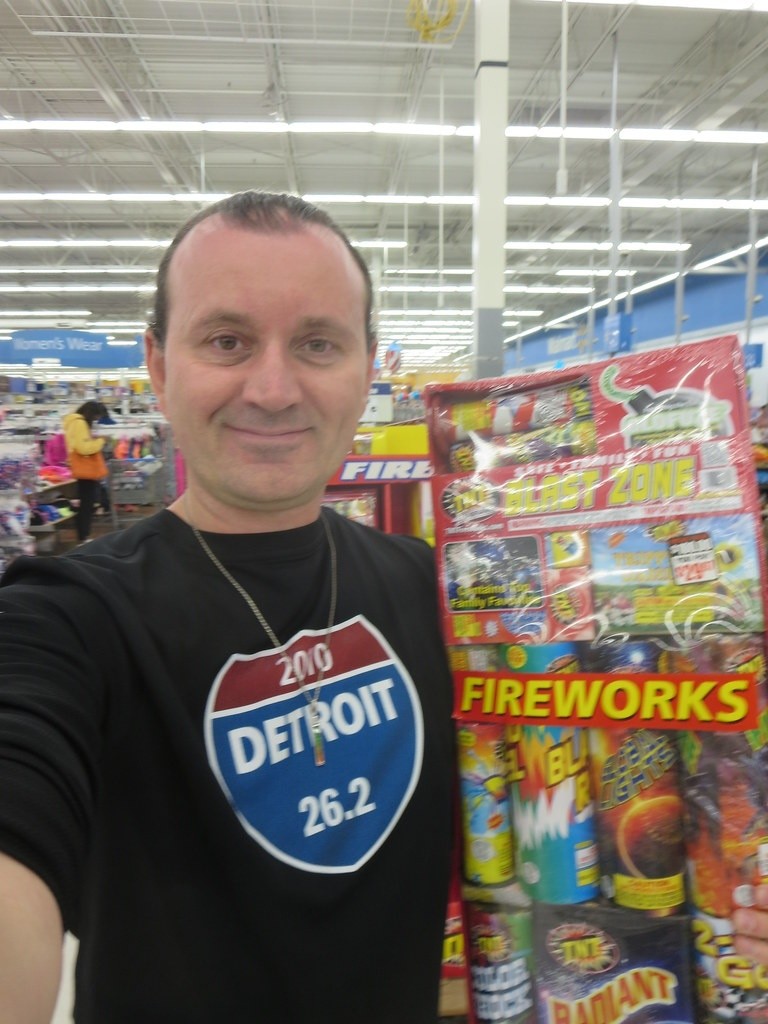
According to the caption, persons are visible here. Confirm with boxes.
[0,190,768,1023]
[62,402,109,543]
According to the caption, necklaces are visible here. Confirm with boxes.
[181,493,338,766]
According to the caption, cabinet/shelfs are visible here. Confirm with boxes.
[17,478,81,532]
